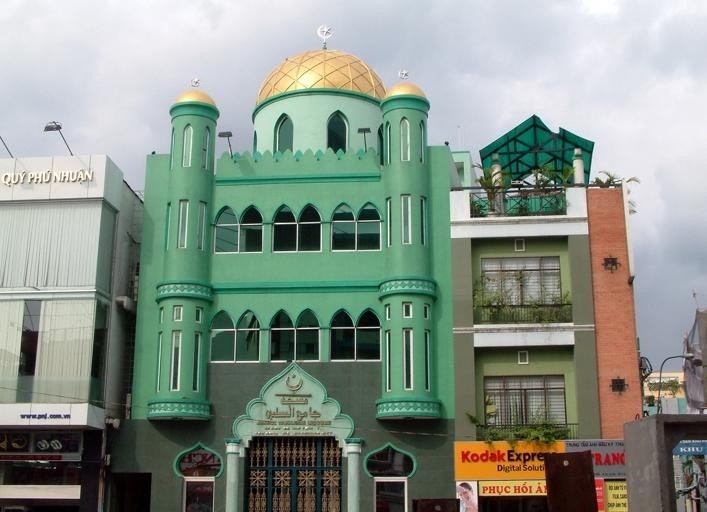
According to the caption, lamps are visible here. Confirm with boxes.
[357,128,372,153]
[44,121,74,157]
[217,131,234,158]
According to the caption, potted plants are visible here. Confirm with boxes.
[472,162,507,217]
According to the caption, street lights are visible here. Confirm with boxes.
[656,352,695,414]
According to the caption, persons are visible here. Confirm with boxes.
[455,482,478,512]
[692,455,706,482]
